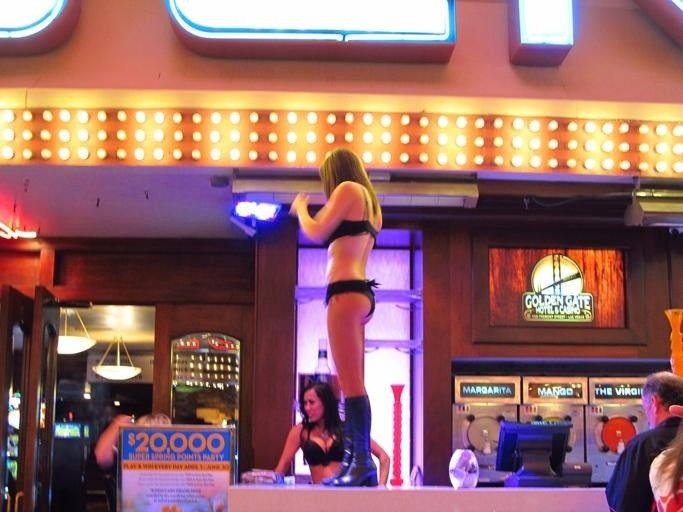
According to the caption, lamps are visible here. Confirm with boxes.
[624,189,683,228]
[231,179,481,210]
[91,332,141,381]
[57,307,96,355]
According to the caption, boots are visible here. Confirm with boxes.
[324,397,378,487]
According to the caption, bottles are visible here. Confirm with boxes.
[313,337,331,388]
[449,448,480,490]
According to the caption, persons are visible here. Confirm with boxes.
[273,381,390,486]
[603,371,682,512]
[93,411,172,473]
[646,402,683,512]
[290,145,384,489]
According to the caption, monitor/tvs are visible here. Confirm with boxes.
[495,420,571,476]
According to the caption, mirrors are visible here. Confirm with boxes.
[168,331,242,491]
